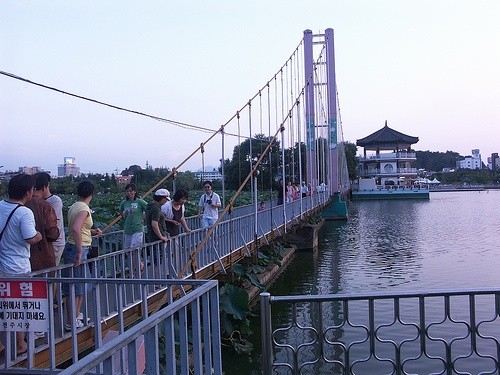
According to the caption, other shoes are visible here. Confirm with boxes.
[33,332,45,340]
[76,313,91,322]
[65,320,84,330]
[141,266,145,275]
[17,344,38,357]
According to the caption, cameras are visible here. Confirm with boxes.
[207,200,212,204]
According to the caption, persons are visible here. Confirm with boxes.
[118,183,193,290]
[0,170,102,359]
[258,178,325,218]
[198,181,221,252]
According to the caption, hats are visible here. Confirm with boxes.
[154,189,171,201]
